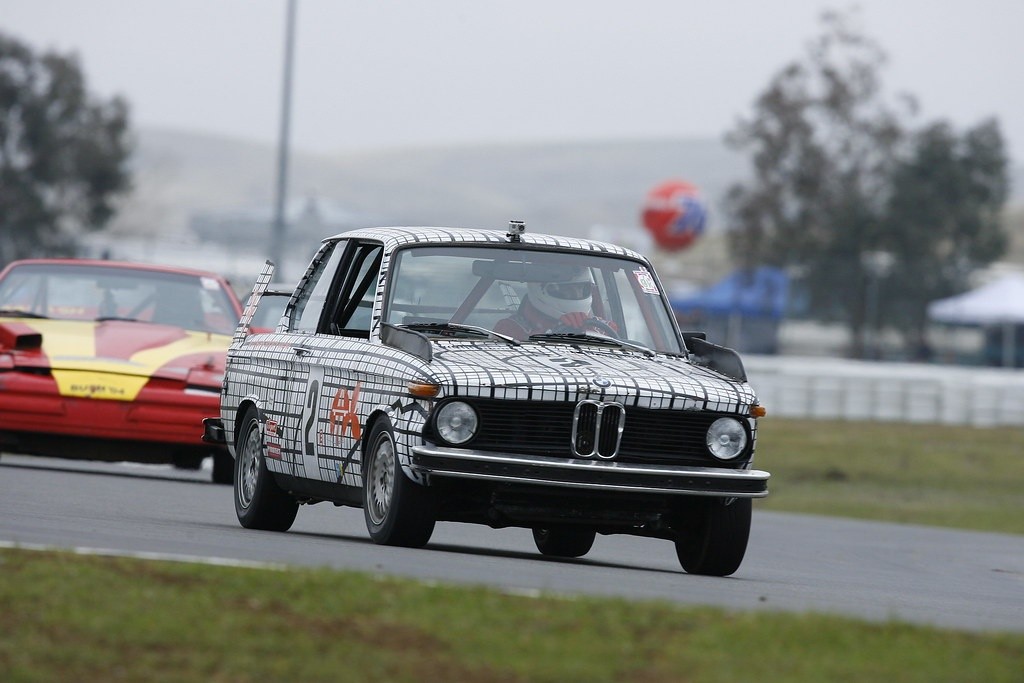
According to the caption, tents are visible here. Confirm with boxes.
[668,264,806,349]
[927,272,1023,370]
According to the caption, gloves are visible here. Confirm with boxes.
[592,317,617,336]
[560,312,588,328]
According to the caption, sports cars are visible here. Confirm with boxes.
[236,285,420,341]
[0,258,278,482]
[202,215,772,578]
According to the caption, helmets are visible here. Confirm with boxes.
[527,254,595,320]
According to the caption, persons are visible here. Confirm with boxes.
[492,264,618,342]
